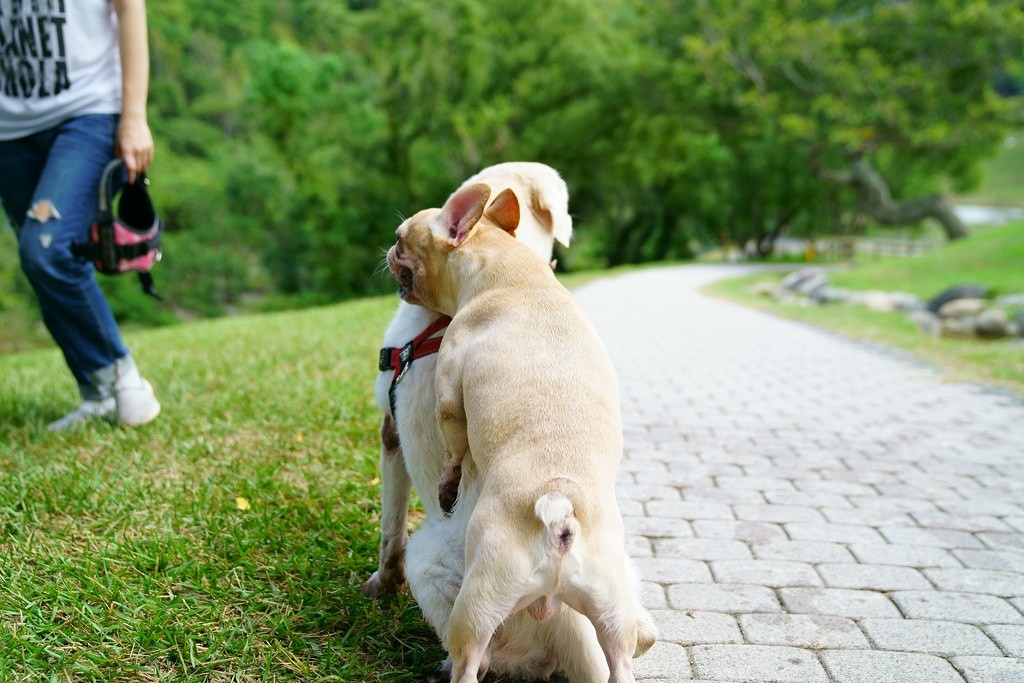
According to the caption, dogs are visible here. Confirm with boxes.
[357,159,659,683]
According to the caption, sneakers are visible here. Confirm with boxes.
[115,374,160,426]
[46,397,117,435]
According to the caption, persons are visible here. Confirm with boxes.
[0,0,161,438]
